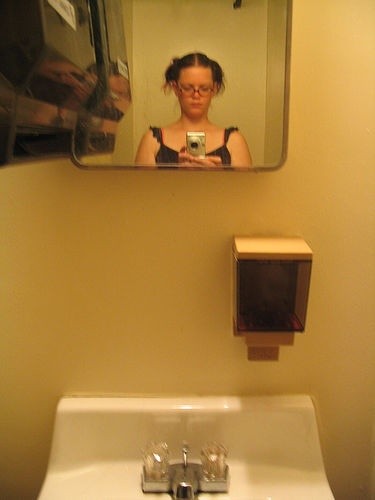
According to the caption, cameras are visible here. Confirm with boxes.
[186,131,207,160]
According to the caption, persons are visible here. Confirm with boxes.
[132,52,254,171]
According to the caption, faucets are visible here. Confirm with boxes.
[167,440,199,500]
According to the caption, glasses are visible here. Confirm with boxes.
[170,80,217,99]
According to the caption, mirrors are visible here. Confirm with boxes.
[64,0,293,173]
